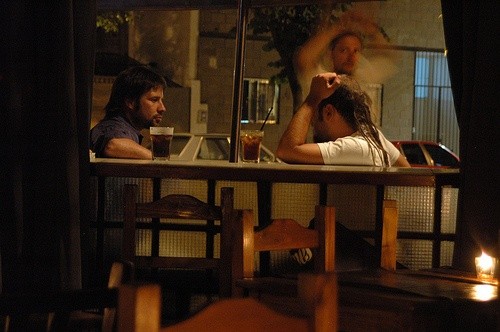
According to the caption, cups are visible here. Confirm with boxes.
[475,258,496,280]
[150,127,173,161]
[240,130,265,163]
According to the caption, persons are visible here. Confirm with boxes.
[275,70,413,167]
[292,9,403,143]
[90,64,165,160]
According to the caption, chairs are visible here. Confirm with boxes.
[0,184,339,332]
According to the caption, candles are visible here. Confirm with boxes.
[474,250,497,279]
